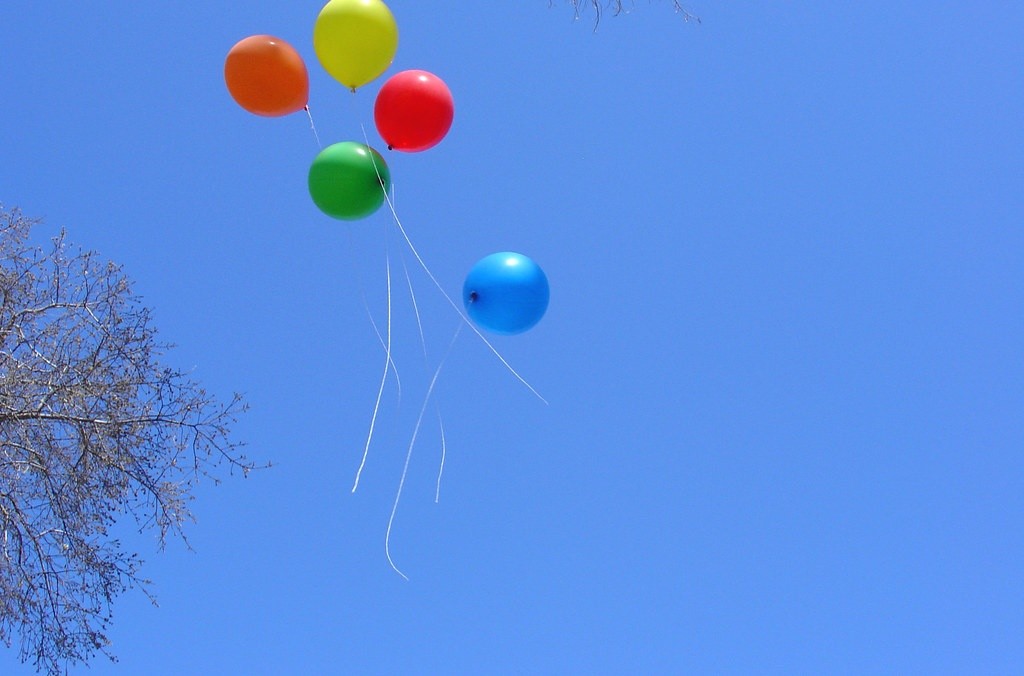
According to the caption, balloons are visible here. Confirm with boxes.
[224,35,310,117]
[463,252,550,336]
[313,0,399,93]
[308,142,392,222]
[374,69,454,153]
